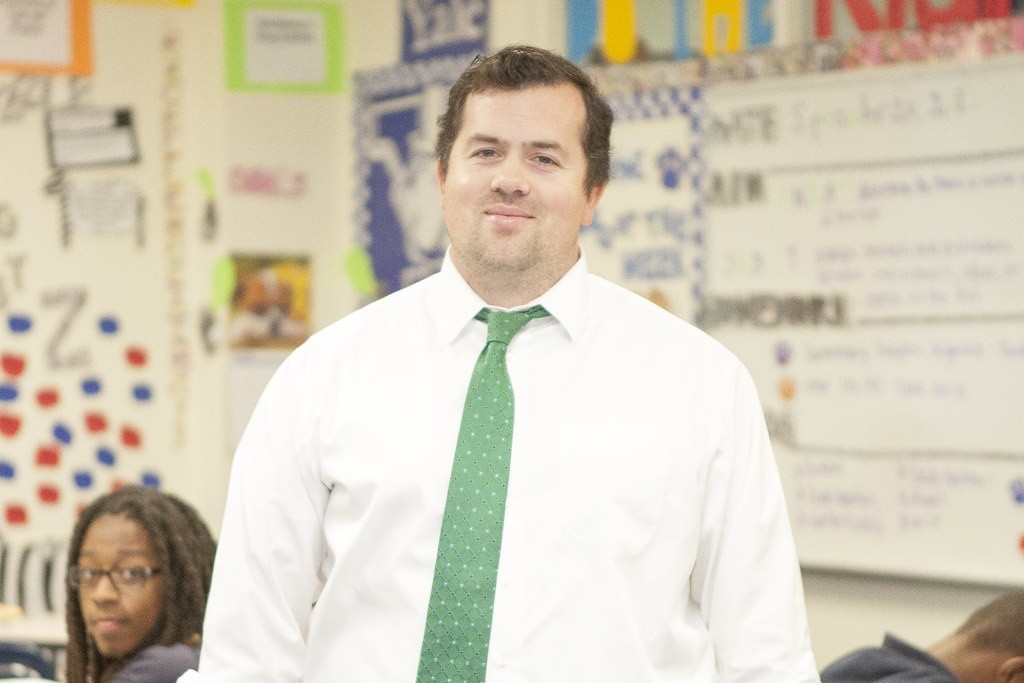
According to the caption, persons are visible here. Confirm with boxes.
[817,588,1023,682]
[64,489,217,683]
[198,44,818,683]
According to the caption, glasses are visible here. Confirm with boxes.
[65,562,163,593]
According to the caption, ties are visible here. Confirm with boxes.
[416,305,551,683]
